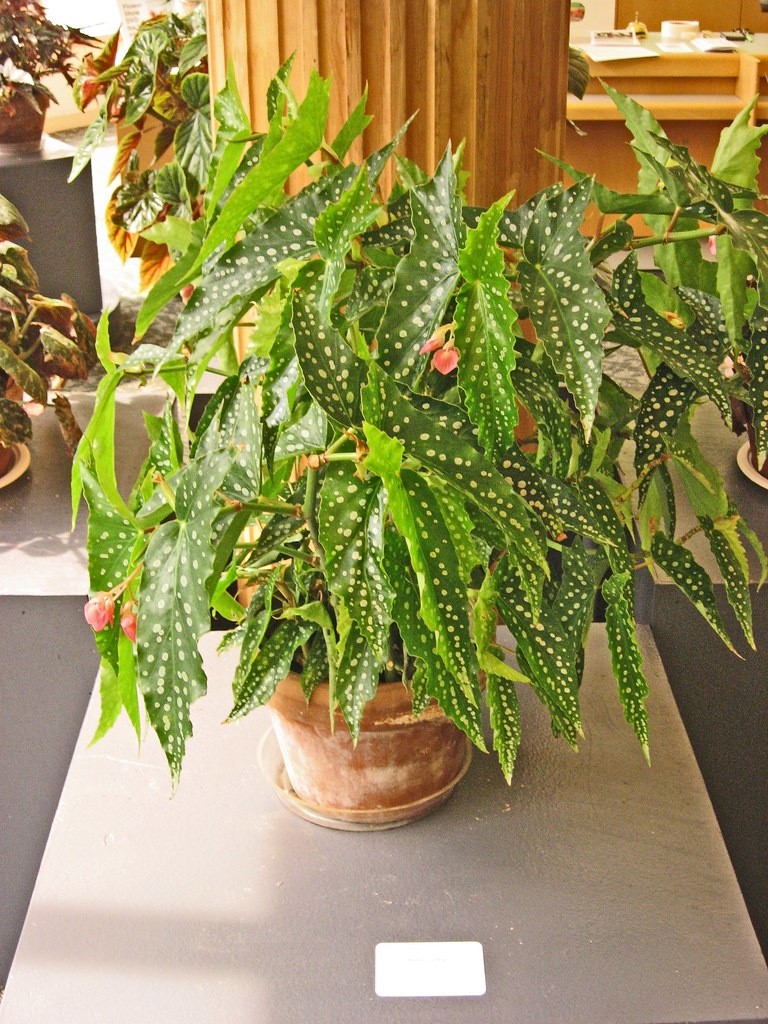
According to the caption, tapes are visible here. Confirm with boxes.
[660,18,700,41]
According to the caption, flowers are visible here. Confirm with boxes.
[57,8,768,799]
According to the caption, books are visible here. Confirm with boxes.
[694,35,737,53]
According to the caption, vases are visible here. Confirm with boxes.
[267,670,486,811]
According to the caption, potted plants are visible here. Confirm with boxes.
[0,0,108,145]
[591,72,768,478]
[0,193,98,475]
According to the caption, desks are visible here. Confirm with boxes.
[562,94,768,239]
[566,29,768,96]
